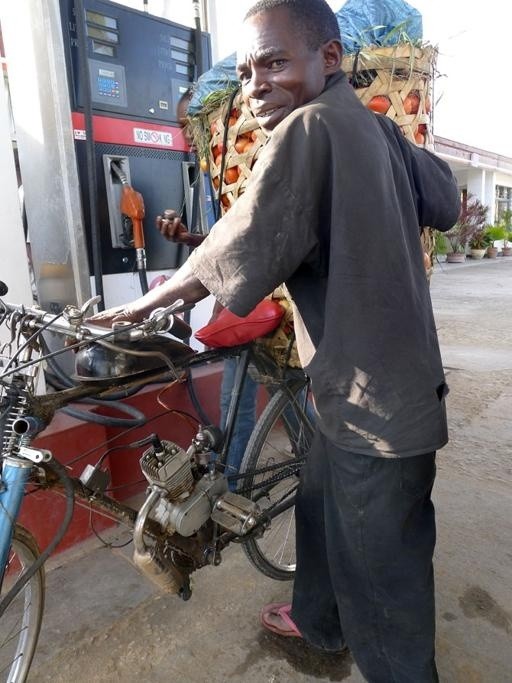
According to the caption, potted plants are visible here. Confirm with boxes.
[431,198,512,264]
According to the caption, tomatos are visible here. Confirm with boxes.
[200,94,434,335]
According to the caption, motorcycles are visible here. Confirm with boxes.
[0,276,316,682]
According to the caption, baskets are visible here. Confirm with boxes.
[189,43,439,367]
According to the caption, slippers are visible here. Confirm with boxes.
[264,601,299,638]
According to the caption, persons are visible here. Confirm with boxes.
[157,86,321,495]
[66,0,461,683]
